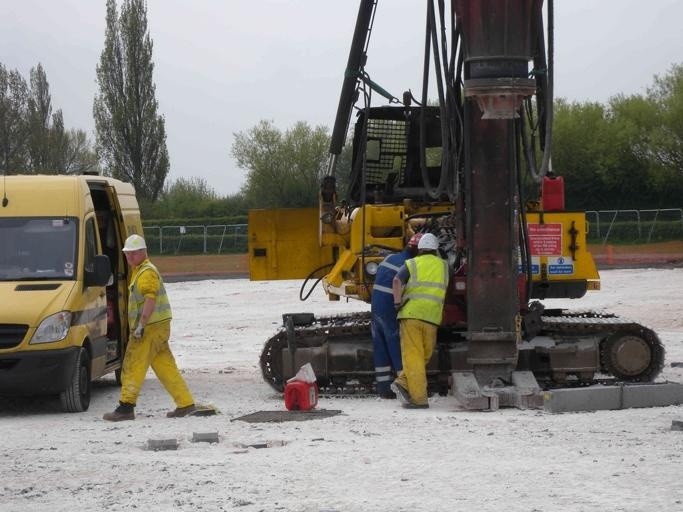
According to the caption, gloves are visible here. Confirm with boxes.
[133,325,144,339]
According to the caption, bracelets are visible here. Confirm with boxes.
[393,302,400,306]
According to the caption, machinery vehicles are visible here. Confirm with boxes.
[247,1,666,412]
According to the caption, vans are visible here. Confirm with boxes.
[0,174,150,413]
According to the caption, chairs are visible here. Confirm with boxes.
[30,211,116,284]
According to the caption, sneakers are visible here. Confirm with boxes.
[381,385,429,409]
[103,410,135,422]
[165,404,196,418]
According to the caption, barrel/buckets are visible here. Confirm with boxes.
[284,383,319,410]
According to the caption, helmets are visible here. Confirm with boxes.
[418,232,439,250]
[408,232,424,246]
[122,233,148,252]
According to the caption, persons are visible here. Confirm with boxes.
[370,233,423,400]
[102,232,196,423]
[388,232,454,409]
